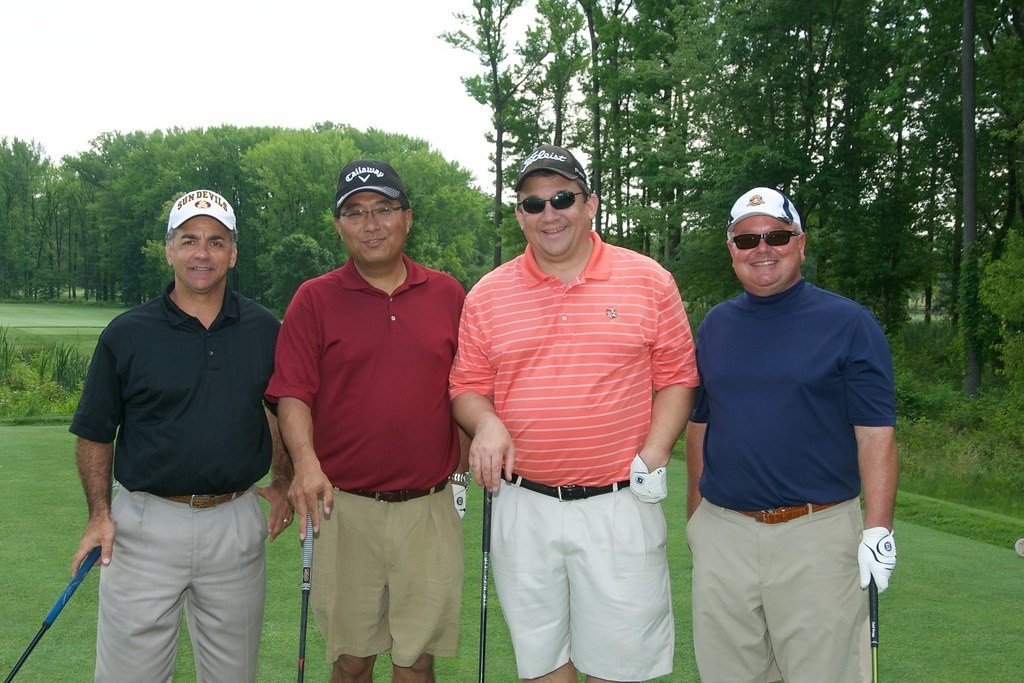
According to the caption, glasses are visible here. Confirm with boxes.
[338,206,406,223]
[730,230,798,250]
[517,192,588,214]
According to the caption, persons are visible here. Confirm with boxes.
[265,159,472,683]
[686,186,898,683]
[68,190,294,683]
[449,145,699,683]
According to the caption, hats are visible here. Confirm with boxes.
[168,189,238,238]
[334,160,410,209]
[515,145,586,194]
[725,187,803,238]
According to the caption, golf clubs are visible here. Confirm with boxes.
[868,574,887,683]
[296,510,315,683]
[478,489,493,683]
[2,543,102,683]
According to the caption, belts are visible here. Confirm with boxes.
[735,505,838,523]
[501,468,630,503]
[153,492,243,509]
[332,478,450,506]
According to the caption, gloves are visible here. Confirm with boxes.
[858,528,896,594]
[452,484,465,520]
[631,453,667,504]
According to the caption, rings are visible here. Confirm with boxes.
[284,519,289,523]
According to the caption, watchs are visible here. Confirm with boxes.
[451,471,473,486]
